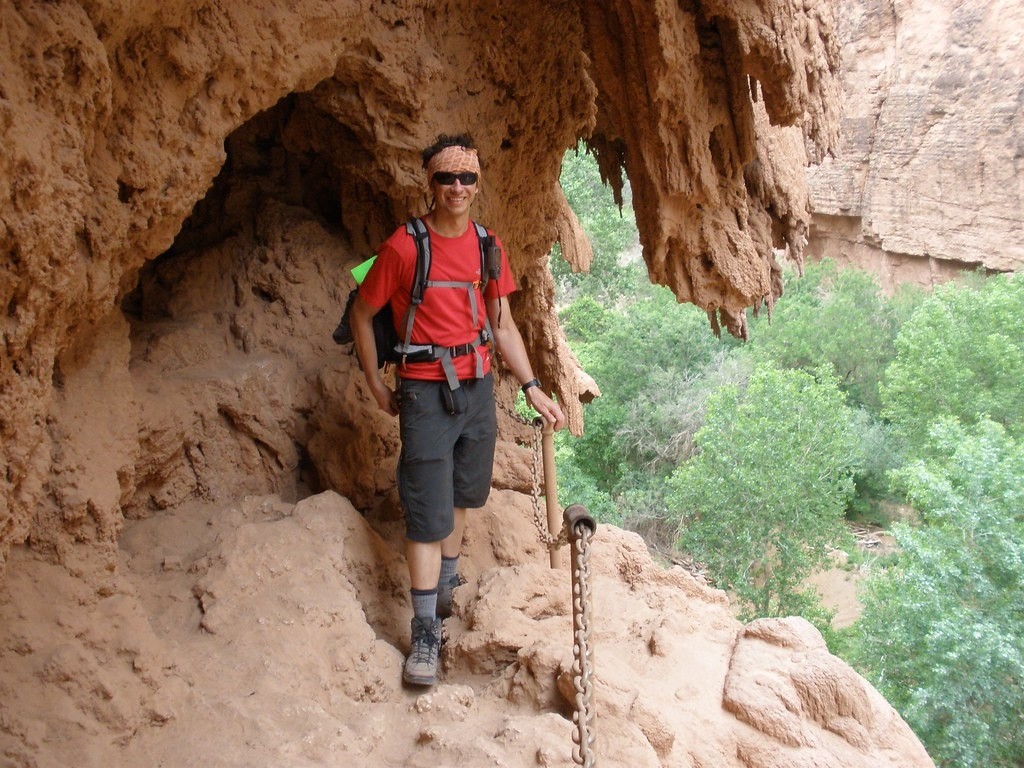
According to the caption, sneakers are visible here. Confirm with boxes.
[402,573,462,686]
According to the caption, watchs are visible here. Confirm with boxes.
[521,379,542,394]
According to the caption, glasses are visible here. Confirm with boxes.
[429,170,478,188]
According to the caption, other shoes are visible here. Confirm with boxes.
[332,286,387,372]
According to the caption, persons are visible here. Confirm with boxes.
[348,133,568,688]
